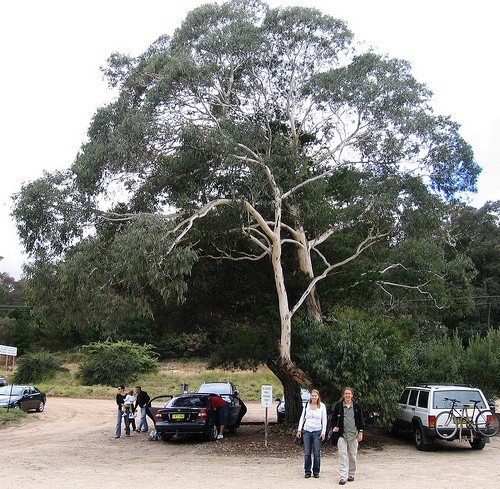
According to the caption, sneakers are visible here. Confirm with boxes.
[217,435,223,439]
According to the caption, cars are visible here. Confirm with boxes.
[275,387,313,422]
[0,378,8,387]
[0,386,46,413]
[145,392,248,443]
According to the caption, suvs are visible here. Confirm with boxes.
[196,381,240,405]
[386,382,493,451]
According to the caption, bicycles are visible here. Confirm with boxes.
[434,398,499,440]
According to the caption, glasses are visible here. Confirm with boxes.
[118,388,122,390]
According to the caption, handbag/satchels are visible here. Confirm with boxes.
[328,426,339,446]
[293,436,303,445]
[129,412,136,419]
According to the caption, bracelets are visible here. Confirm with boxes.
[359,429,363,432]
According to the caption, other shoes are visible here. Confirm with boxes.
[305,474,311,478]
[314,474,319,477]
[114,426,148,438]
[339,480,346,485]
[348,476,354,480]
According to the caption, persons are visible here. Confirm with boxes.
[112,385,132,438]
[295,388,328,479]
[120,389,135,418]
[329,386,365,484]
[133,385,151,433]
[209,395,230,440]
